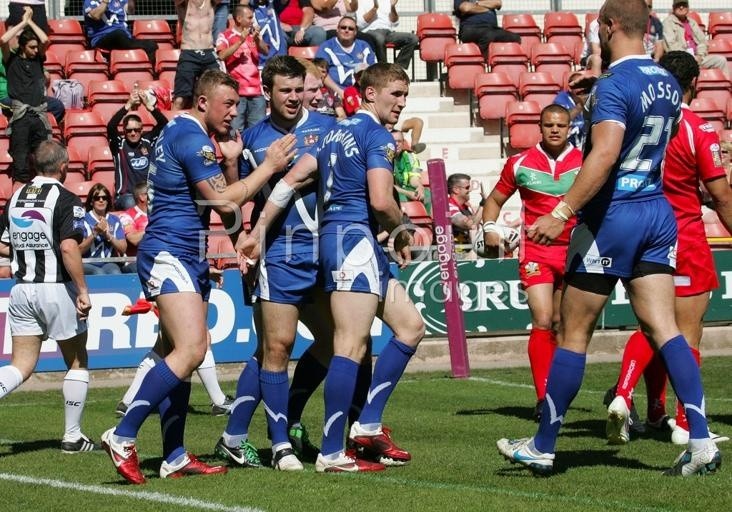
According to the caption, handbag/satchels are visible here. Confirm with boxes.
[52,79,84,109]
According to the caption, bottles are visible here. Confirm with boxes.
[133,82,141,104]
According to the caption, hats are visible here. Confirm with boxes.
[673,0,688,8]
[354,63,369,74]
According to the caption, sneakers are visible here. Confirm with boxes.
[101,427,147,485]
[605,396,633,445]
[345,438,406,466]
[348,421,411,461]
[671,425,730,445]
[214,436,263,472]
[411,143,426,154]
[287,424,321,464]
[160,451,228,480]
[496,438,556,473]
[115,399,131,415]
[211,395,236,418]
[644,414,674,435]
[534,400,544,422]
[315,449,386,472]
[660,442,721,480]
[271,442,304,471]
[60,435,106,455]
[603,388,648,440]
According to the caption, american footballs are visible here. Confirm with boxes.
[473,226,521,258]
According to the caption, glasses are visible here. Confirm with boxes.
[92,195,109,201]
[722,151,731,153]
[461,186,470,190]
[341,26,354,31]
[125,127,142,133]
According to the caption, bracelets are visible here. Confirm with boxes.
[550,200,577,223]
[107,235,113,243]
[146,101,152,112]
[102,1,111,6]
[482,221,497,233]
[478,199,488,206]
[238,177,249,201]
[300,27,306,33]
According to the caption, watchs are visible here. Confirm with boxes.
[476,1,479,6]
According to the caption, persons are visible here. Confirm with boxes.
[213,56,335,469]
[313,59,330,110]
[235,62,426,473]
[114,266,236,419]
[553,72,587,155]
[663,0,730,80]
[0,5,51,185]
[341,68,363,122]
[454,0,522,64]
[386,130,433,219]
[308,0,358,41]
[215,4,273,137]
[313,16,382,102]
[6,0,55,37]
[271,0,326,48]
[118,183,150,274]
[76,184,128,276]
[495,1,723,478]
[216,54,409,471]
[0,215,12,278]
[212,0,233,45]
[445,172,487,242]
[170,0,223,112]
[578,15,604,79]
[703,139,732,209]
[0,140,105,454]
[353,0,420,72]
[603,349,691,447]
[481,103,583,421]
[83,0,158,70]
[641,0,664,61]
[1,16,66,124]
[99,67,300,485]
[605,49,732,446]
